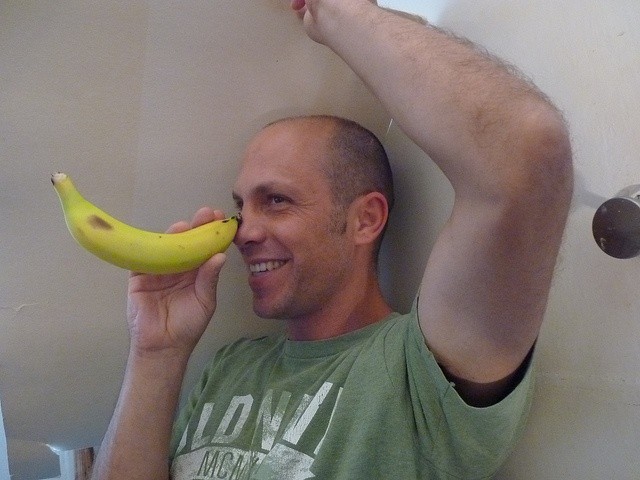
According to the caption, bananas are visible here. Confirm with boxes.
[49,172,239,276]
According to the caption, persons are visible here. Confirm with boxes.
[90,0,575,480]
